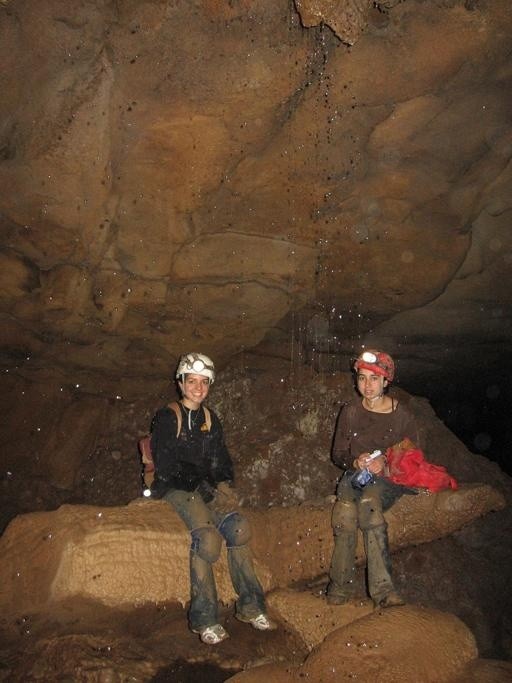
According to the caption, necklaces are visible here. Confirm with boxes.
[182,400,201,427]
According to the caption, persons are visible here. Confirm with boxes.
[326,350,425,607]
[151,353,277,644]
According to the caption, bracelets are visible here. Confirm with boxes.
[381,454,389,467]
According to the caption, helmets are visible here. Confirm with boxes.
[176,352,216,385]
[353,348,395,382]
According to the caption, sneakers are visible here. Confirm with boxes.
[190,622,230,646]
[235,610,277,631]
[379,592,405,608]
[327,581,352,604]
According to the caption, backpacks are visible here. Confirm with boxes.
[139,401,212,489]
